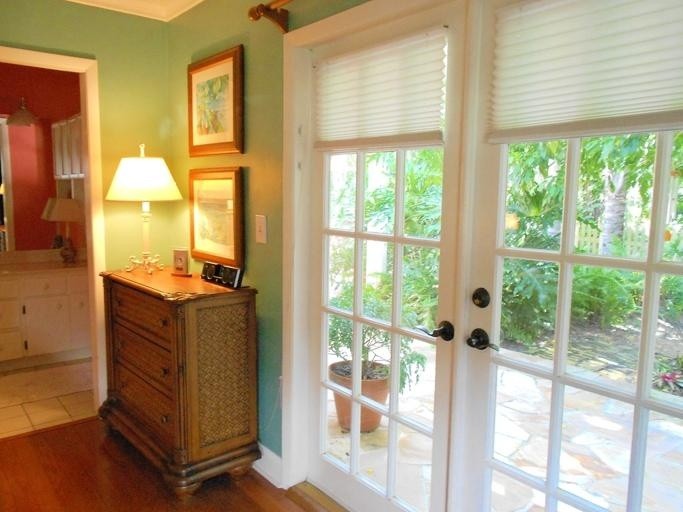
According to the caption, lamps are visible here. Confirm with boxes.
[50,198,85,266]
[5,96,37,128]
[40,199,64,249]
[103,144,185,276]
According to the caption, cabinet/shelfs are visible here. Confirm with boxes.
[24,268,92,369]
[67,113,84,181]
[0,271,26,375]
[50,119,67,180]
[96,264,261,503]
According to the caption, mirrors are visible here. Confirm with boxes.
[0,115,57,254]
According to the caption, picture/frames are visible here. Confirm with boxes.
[186,44,245,158]
[185,163,247,272]
[169,249,190,276]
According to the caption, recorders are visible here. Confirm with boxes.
[201,261,242,288]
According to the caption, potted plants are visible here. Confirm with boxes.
[326,271,428,434]
